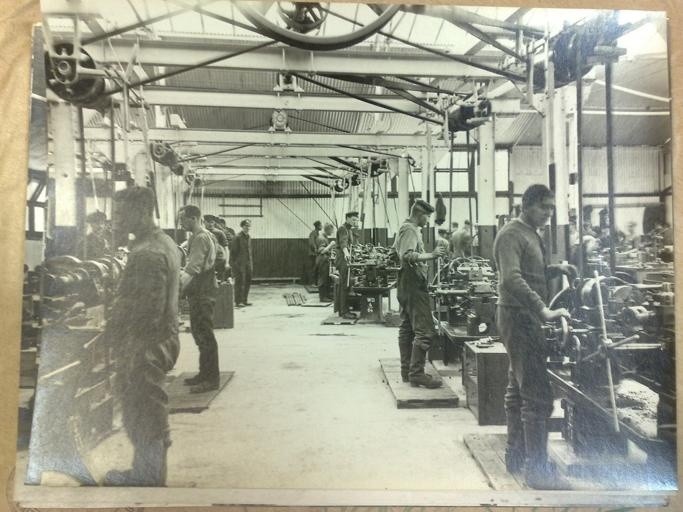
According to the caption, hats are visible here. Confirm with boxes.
[347,212,359,217]
[416,200,434,213]
[314,221,320,226]
[86,212,106,223]
[241,219,251,227]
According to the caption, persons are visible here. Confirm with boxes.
[103,188,182,488]
[79,212,113,259]
[492,183,579,491]
[179,205,219,393]
[390,200,446,388]
[301,208,361,320]
[433,220,474,259]
[229,218,253,307]
[200,214,235,284]
[569,216,674,276]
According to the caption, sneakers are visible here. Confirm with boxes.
[403,373,442,388]
[506,454,571,490]
[342,312,356,319]
[104,469,166,485]
[184,375,219,392]
[310,283,317,287]
[236,301,251,307]
